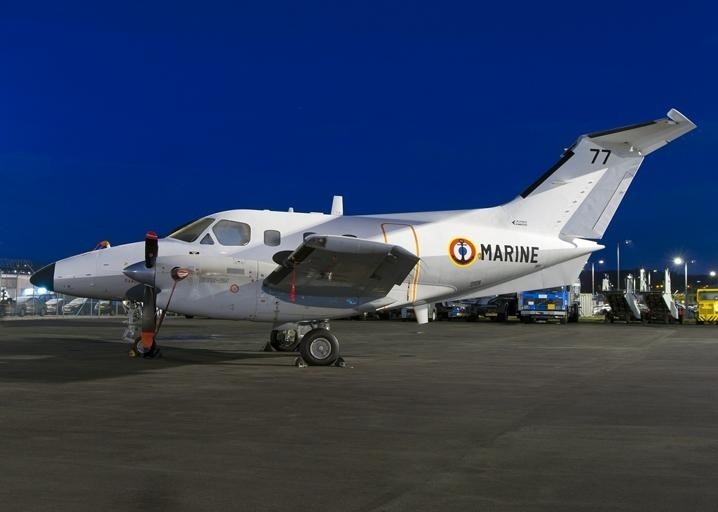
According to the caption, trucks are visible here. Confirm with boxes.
[520,279,580,324]
[694,288,718,325]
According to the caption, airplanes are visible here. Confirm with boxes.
[30,108,698,367]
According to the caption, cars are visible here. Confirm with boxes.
[604,304,651,323]
[645,302,687,325]
[0,297,129,317]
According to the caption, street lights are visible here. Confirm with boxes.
[674,258,696,293]
[615,238,632,290]
[586,260,604,295]
